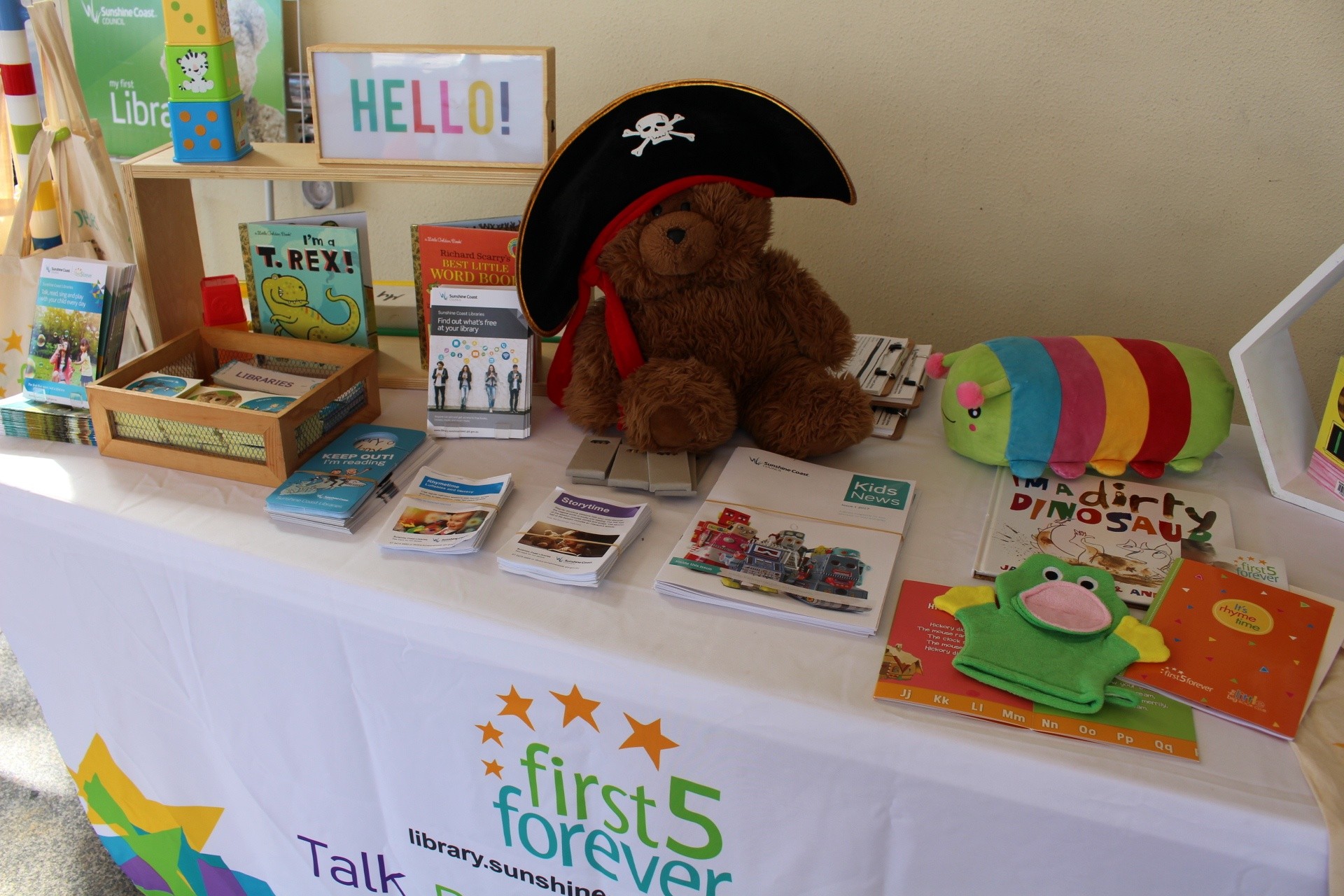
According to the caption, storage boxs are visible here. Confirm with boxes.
[86,325,381,489]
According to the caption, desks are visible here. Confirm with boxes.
[0,350,1344,896]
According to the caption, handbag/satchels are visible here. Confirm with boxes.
[1,127,145,398]
[28,1,136,264]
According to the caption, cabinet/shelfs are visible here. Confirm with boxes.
[121,140,545,389]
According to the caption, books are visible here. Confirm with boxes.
[0,256,366,463]
[272,418,1334,767]
[428,286,534,415]
[239,212,526,372]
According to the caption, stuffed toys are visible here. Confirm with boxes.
[925,337,1235,478]
[514,76,874,456]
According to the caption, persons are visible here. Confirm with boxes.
[484,364,498,413]
[50,338,75,385]
[509,364,522,414]
[71,337,94,385]
[457,364,473,410]
[432,361,449,410]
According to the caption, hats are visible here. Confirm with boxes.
[514,79,857,338]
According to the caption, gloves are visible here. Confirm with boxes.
[931,553,1171,714]
